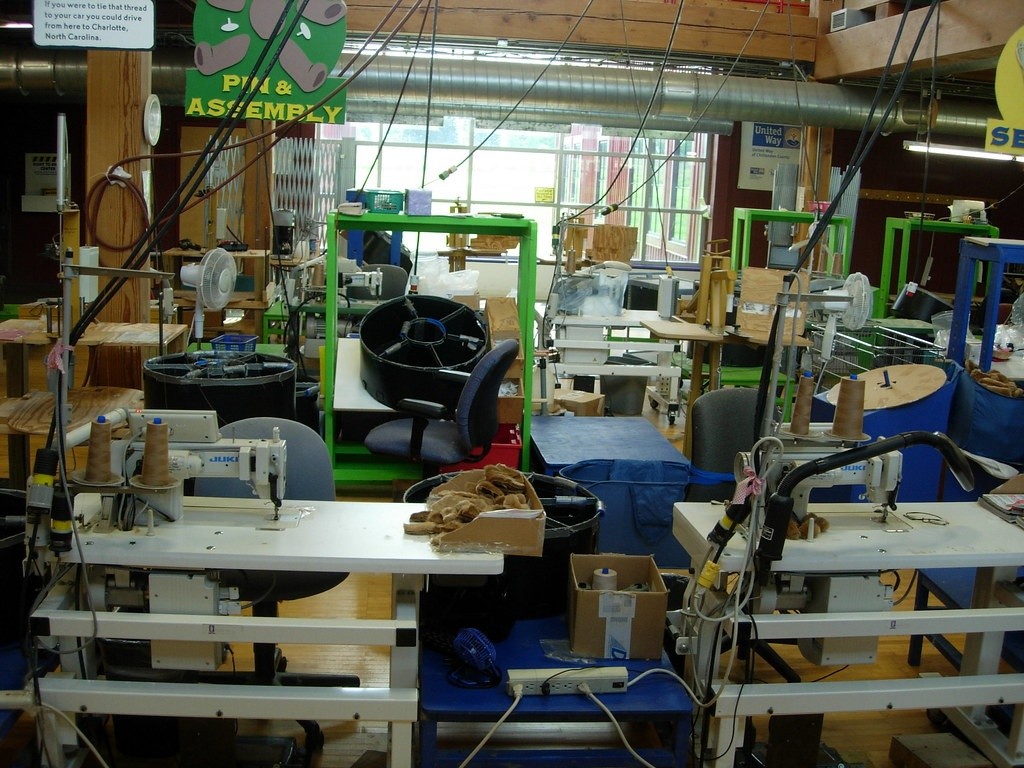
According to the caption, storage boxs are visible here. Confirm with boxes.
[405,190,432,216]
[427,464,547,556]
[567,554,669,660]
[440,297,605,474]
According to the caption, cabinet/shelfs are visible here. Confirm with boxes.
[162,247,270,347]
[269,256,325,344]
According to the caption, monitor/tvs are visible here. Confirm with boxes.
[767,244,801,271]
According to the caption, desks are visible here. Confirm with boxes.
[32,492,503,768]
[672,502,1024,768]
[262,300,306,345]
[531,304,682,428]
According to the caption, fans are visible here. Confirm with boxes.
[451,627,501,684]
[817,272,872,394]
[180,247,238,351]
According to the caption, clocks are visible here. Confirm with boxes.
[144,94,162,145]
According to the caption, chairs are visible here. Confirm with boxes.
[365,338,520,503]
[182,416,361,750]
[683,388,803,683]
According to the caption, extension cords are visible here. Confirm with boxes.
[504,667,628,695]
